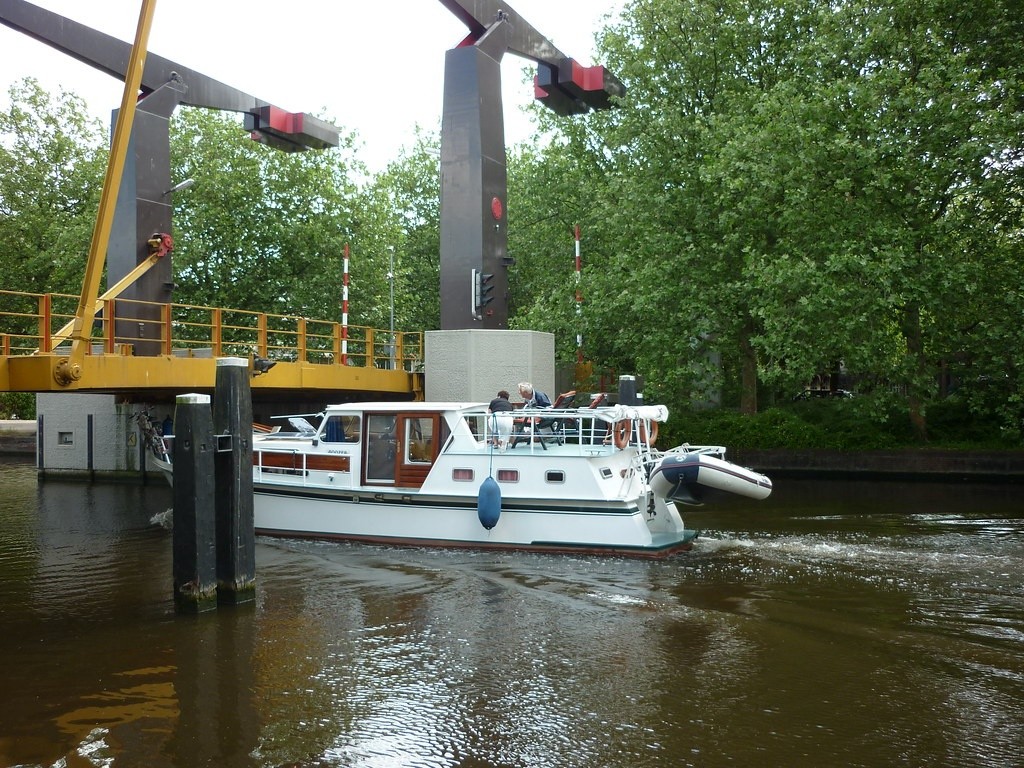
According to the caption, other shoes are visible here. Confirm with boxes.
[493,444,498,449]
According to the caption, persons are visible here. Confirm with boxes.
[486,391,516,450]
[518,382,552,427]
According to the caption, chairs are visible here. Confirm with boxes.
[511,391,576,450]
[554,393,605,445]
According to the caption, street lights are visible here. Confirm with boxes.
[388,245,395,369]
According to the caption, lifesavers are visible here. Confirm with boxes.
[613,418,632,449]
[641,418,659,445]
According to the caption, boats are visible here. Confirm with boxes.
[131,375,772,557]
